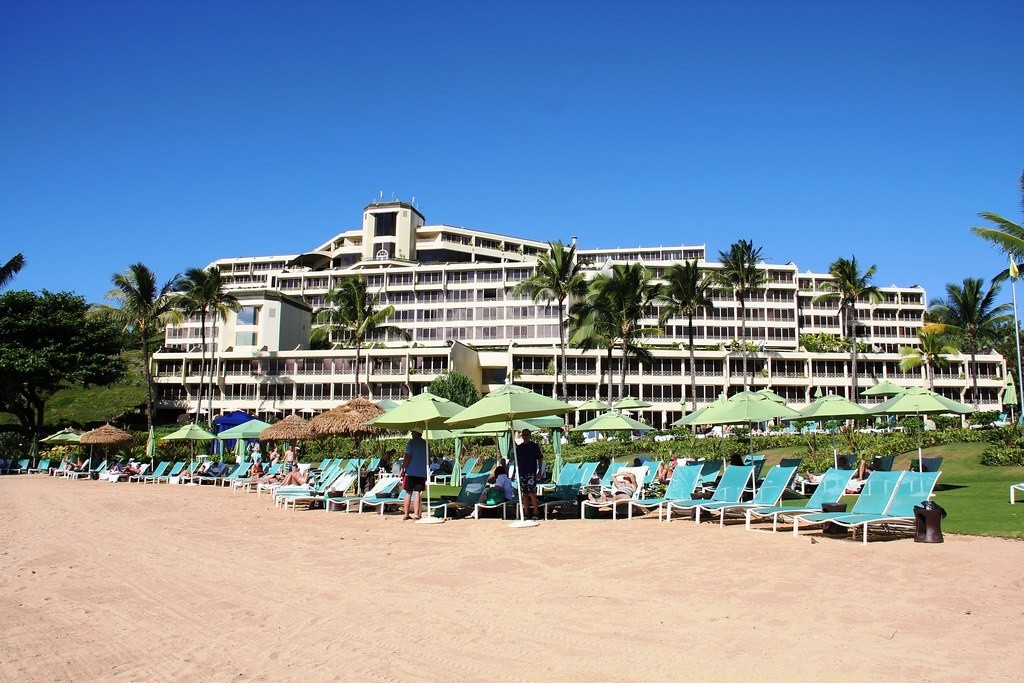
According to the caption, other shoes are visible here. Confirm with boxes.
[464,515,475,519]
[524,515,530,519]
[532,515,538,520]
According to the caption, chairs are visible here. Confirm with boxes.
[0,452,944,542]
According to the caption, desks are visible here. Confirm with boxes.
[912,506,944,544]
[376,492,392,513]
[309,491,324,510]
[822,503,848,534]
[689,492,704,519]
[328,491,344,511]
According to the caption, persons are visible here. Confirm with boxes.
[658,456,678,483]
[401,427,430,520]
[202,462,219,476]
[464,448,520,518]
[537,454,546,506]
[178,465,206,481]
[516,429,543,520]
[430,447,455,482]
[63,446,140,479]
[375,448,396,471]
[246,444,309,485]
[588,472,638,502]
[596,455,611,480]
[793,459,874,494]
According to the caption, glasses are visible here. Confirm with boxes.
[523,434,529,436]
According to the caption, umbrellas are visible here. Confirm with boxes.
[569,412,655,462]
[39,426,83,462]
[1002,374,1018,424]
[306,397,406,454]
[574,398,610,418]
[669,370,979,472]
[147,427,156,472]
[29,432,40,469]
[160,424,218,465]
[259,414,319,463]
[80,422,133,468]
[356,383,576,440]
[614,395,653,419]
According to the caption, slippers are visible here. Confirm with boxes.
[414,516,422,520]
[403,516,413,520]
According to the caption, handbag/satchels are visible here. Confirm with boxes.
[309,491,324,509]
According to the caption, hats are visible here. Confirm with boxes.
[295,447,300,450]
[252,446,259,451]
[411,426,423,434]
[669,456,677,461]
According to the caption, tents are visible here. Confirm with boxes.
[210,410,285,453]
